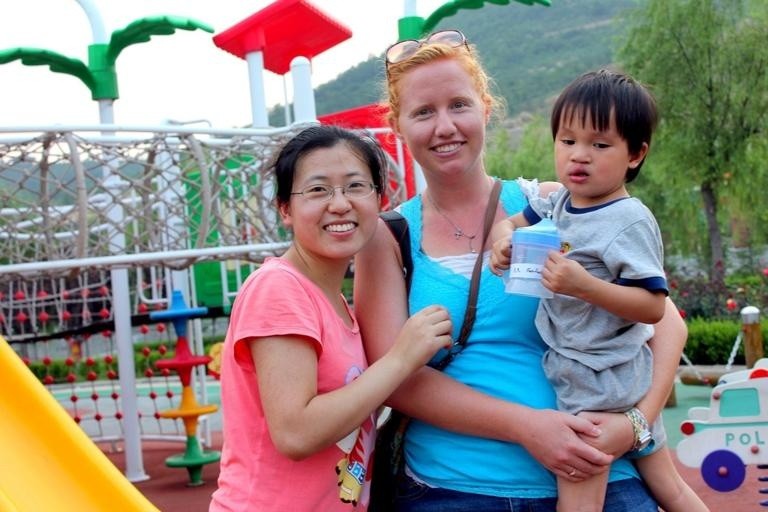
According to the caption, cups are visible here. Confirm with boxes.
[504,218,562,300]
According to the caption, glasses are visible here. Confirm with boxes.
[385,29,470,81]
[290,181,379,203]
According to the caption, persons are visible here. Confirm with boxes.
[207,128,453,512]
[354,41,689,512]
[490,68,710,511]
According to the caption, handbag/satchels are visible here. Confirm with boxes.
[365,408,413,512]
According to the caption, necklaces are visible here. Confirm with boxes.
[425,175,493,253]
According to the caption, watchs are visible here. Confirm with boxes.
[625,406,652,452]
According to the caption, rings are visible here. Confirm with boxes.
[569,469,576,476]
